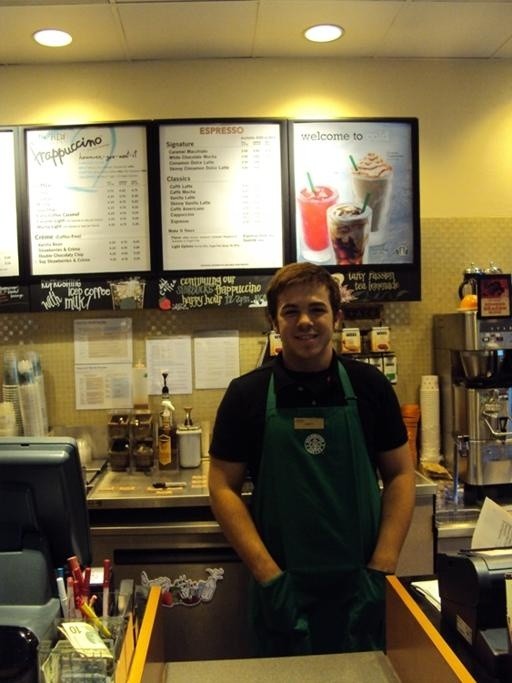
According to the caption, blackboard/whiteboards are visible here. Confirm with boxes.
[26,271,429,311]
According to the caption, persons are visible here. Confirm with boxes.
[208,263,415,656]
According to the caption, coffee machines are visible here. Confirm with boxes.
[431,312,511,510]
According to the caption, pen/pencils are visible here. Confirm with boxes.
[152,482,189,488]
[56,556,113,641]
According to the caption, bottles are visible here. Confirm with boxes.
[158,371,177,471]
[462,260,506,311]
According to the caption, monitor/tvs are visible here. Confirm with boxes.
[0,436,92,586]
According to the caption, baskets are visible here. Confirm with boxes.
[36,591,132,682]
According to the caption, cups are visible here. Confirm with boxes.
[1,347,51,437]
[352,169,392,245]
[401,373,441,464]
[321,205,374,263]
[296,184,342,253]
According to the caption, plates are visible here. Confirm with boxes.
[301,238,334,265]
